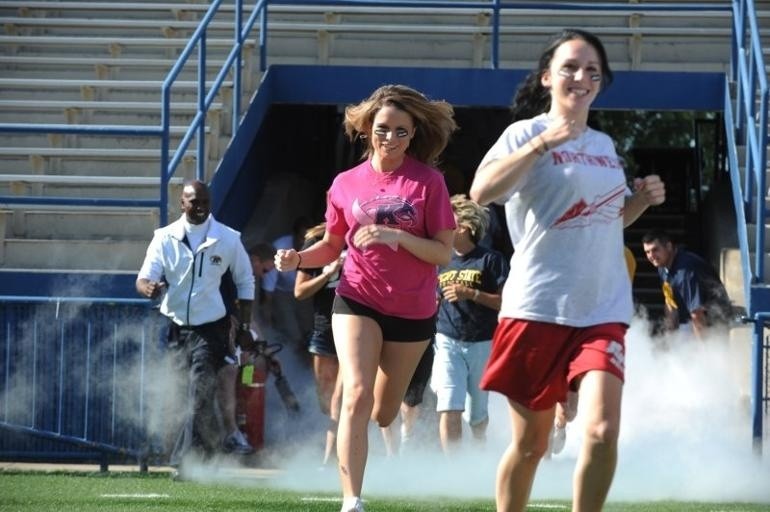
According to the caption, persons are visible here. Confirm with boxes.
[469,28,668,510]
[538,244,636,463]
[134,180,256,481]
[428,195,509,451]
[209,220,438,457]
[642,228,730,345]
[274,84,458,511]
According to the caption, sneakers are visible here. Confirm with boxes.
[399,422,414,455]
[223,429,252,455]
[552,420,566,454]
[342,495,364,512]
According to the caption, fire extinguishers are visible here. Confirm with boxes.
[235,339,282,451]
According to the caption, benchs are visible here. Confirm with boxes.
[0,0,770,275]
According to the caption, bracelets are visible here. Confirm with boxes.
[297,253,301,271]
